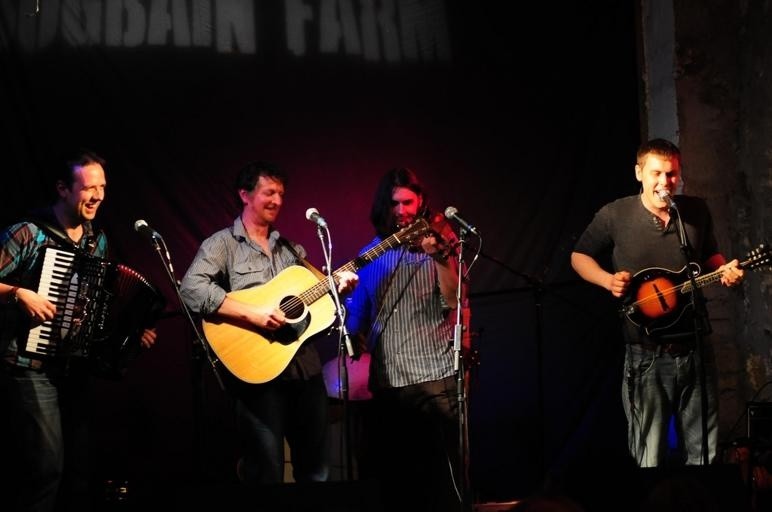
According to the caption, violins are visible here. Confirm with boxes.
[409,212,459,260]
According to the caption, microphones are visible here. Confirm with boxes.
[444,207,481,235]
[658,190,676,208]
[135,219,162,238]
[305,208,328,227]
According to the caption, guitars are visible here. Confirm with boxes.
[621,244,772,329]
[202,219,429,384]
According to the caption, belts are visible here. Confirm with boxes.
[646,340,689,353]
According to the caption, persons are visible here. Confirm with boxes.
[342,165,469,512]
[0,152,157,512]
[180,164,359,483]
[571,138,746,468]
